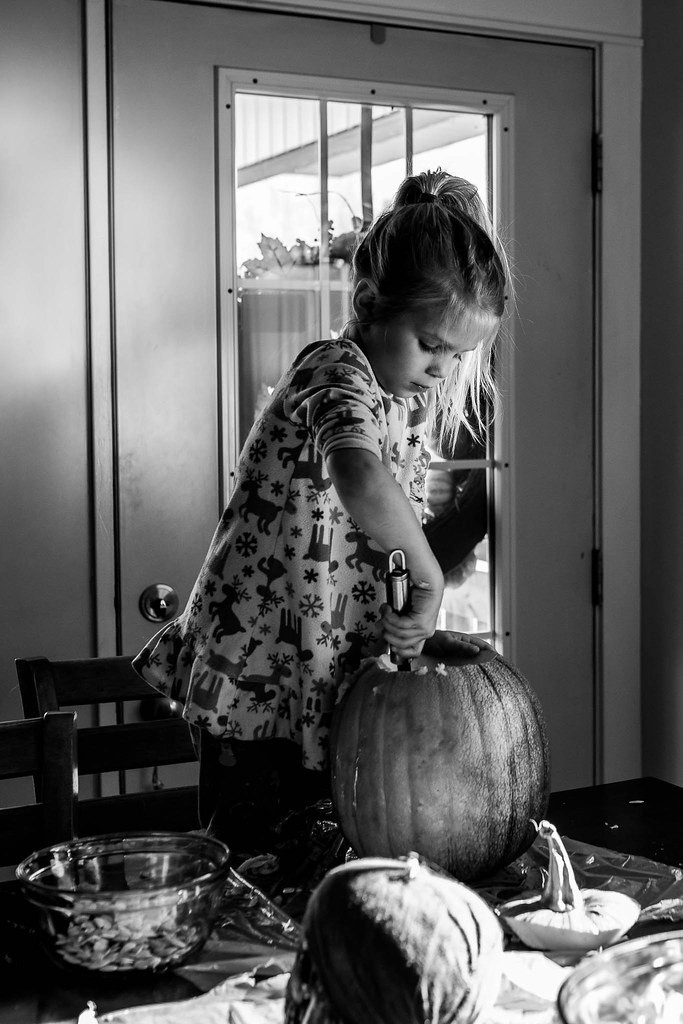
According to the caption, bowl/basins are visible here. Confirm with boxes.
[14,831,230,974]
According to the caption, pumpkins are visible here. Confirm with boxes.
[303,857,506,1024]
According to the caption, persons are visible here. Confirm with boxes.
[131,169,516,837]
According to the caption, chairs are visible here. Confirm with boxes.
[0,711,79,908]
[16,657,201,833]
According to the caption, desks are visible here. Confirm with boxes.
[0,776,683,1024]
